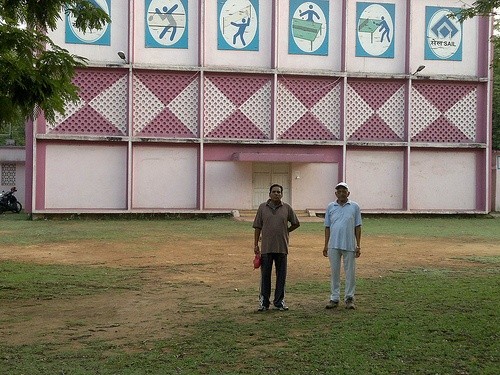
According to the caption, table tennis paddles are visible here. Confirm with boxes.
[253,252,261,269]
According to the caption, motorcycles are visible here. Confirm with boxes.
[0,187,22,215]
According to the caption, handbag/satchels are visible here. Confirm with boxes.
[253,254,261,269]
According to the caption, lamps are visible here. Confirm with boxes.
[117,51,129,64]
[412,65,425,76]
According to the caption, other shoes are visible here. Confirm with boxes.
[345,302,355,309]
[275,300,289,310]
[257,305,270,311]
[326,300,340,308]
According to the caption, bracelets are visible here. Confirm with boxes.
[253,246,259,248]
[356,246,361,250]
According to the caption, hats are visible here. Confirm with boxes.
[335,182,348,189]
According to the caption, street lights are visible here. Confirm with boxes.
[412,65,425,76]
[118,51,129,64]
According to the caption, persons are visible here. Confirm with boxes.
[322,182,362,309]
[252,184,300,311]
